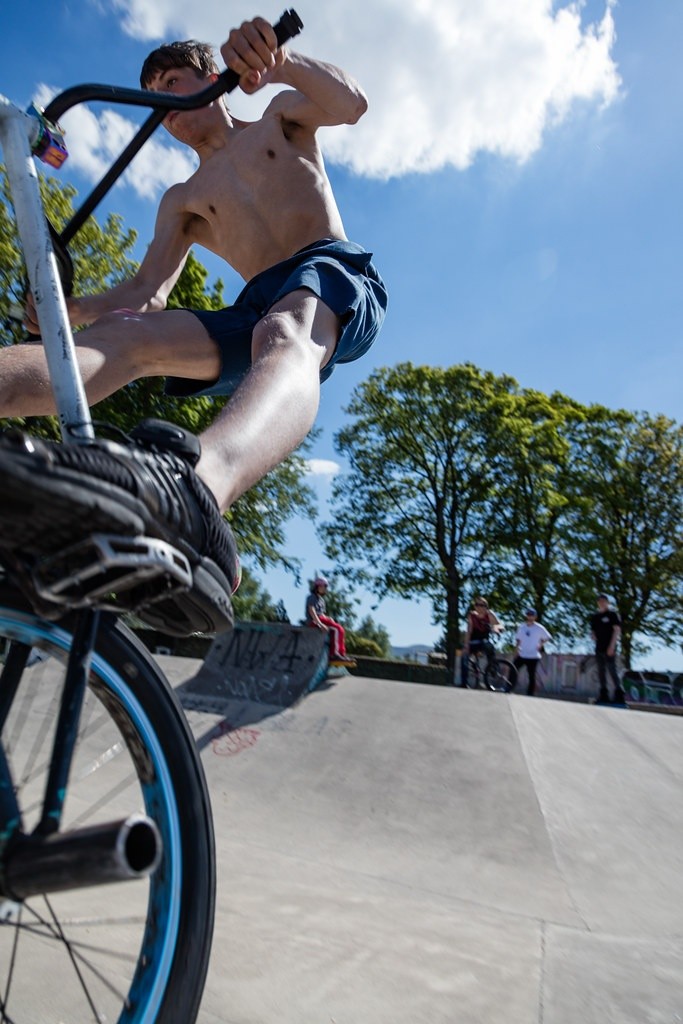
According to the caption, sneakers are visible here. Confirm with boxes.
[0,417,243,636]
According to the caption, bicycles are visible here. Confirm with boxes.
[0,0,311,1024]
[456,628,519,694]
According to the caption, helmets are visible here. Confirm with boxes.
[315,577,328,586]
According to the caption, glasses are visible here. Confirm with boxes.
[475,602,485,607]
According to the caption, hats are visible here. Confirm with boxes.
[525,607,538,615]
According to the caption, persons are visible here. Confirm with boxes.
[0,14,388,636]
[589,595,625,704]
[306,578,357,667]
[461,597,505,690]
[500,608,552,695]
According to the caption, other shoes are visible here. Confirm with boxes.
[457,682,470,688]
[342,654,356,667]
[331,651,349,667]
[613,687,628,708]
[593,686,611,708]
